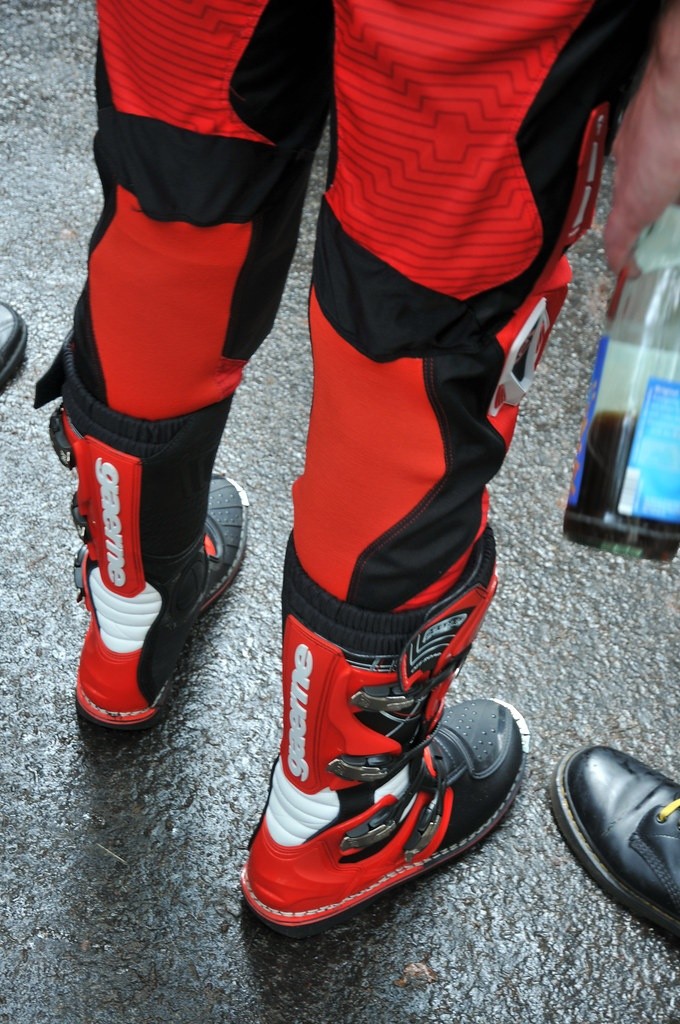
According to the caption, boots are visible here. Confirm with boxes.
[33,332,252,734]
[237,531,530,941]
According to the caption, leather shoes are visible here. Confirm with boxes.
[547,746,680,943]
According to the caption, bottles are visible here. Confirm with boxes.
[561,203,680,564]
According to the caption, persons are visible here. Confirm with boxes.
[34,0,662,936]
[550,0,680,934]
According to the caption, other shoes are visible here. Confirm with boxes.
[0,300,29,389]
